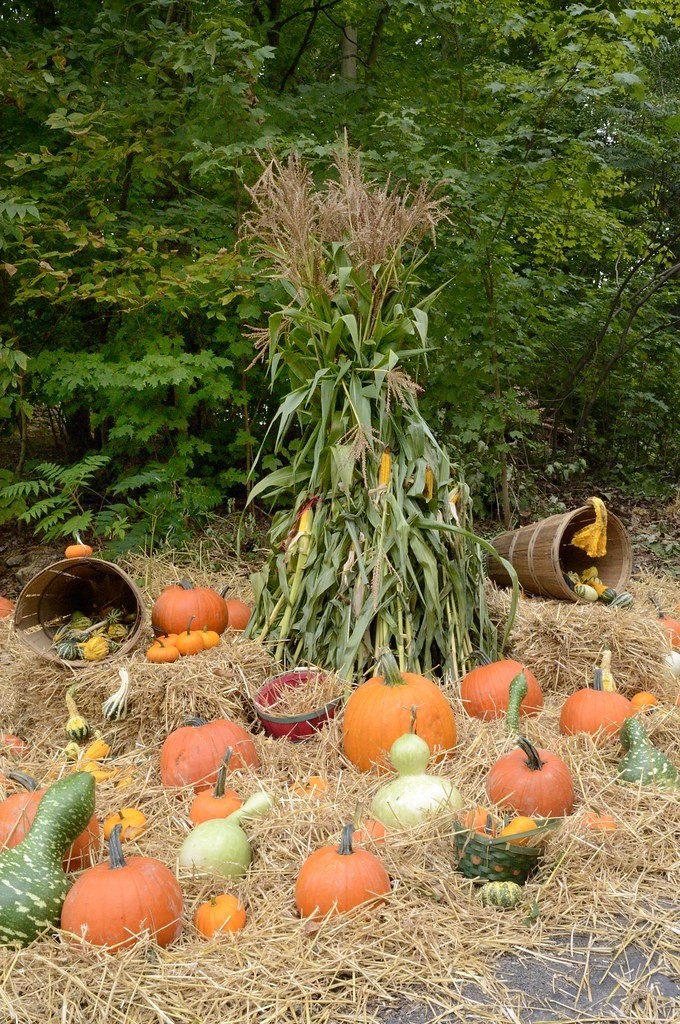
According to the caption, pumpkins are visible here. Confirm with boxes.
[0,544,679,962]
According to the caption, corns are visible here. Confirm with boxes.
[298,448,460,534]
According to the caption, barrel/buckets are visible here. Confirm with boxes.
[254,665,337,742]
